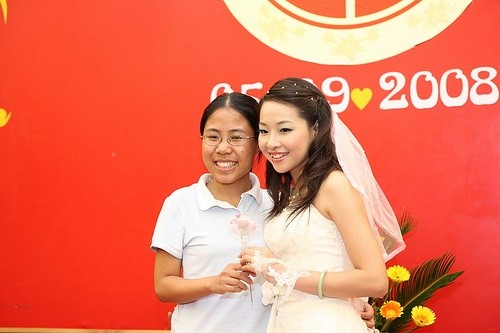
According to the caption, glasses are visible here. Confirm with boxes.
[199,132,257,147]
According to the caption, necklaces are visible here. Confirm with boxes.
[287,184,312,201]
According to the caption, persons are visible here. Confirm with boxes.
[149,92,375,333]
[238,77,407,333]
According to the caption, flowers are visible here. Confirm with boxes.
[227,212,260,304]
[369,212,464,333]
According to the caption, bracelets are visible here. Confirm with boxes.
[317,271,328,300]
